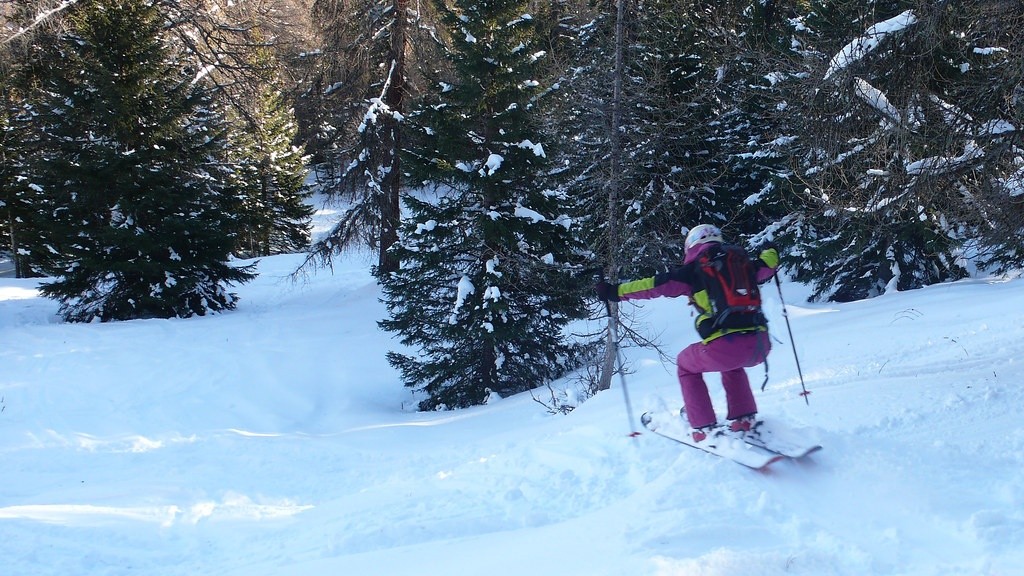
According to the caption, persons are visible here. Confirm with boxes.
[598,224,780,442]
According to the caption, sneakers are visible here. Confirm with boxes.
[721,417,764,440]
[692,424,724,447]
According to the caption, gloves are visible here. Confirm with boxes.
[595,281,622,303]
[757,241,777,257]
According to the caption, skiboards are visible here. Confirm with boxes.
[640,405,823,470]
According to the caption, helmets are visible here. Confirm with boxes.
[685,224,723,253]
